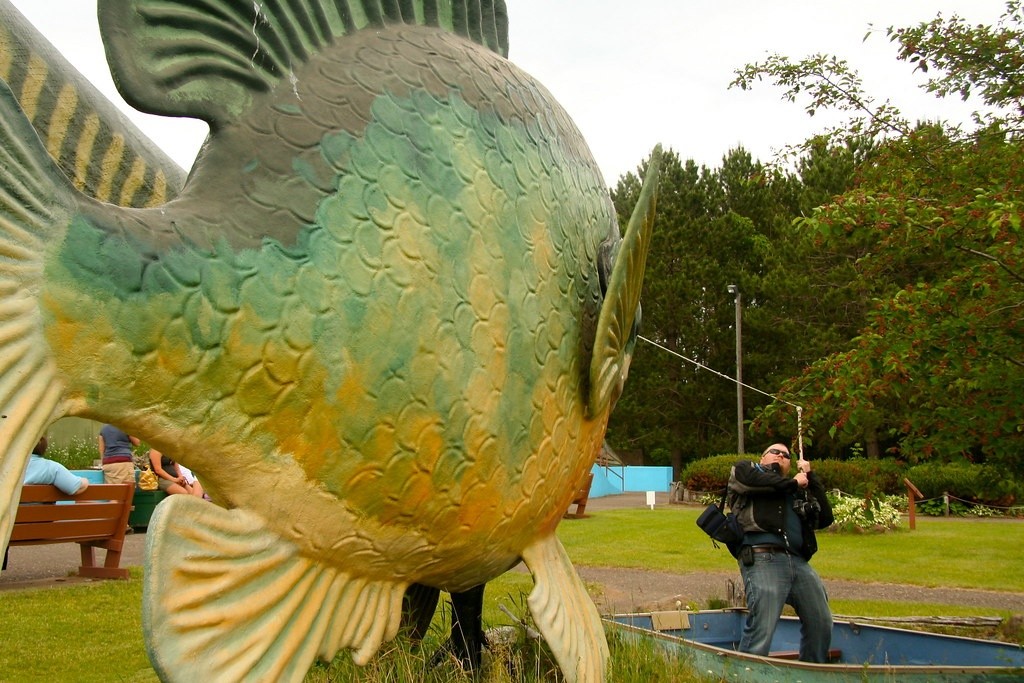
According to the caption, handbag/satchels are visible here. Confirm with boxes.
[138,465,158,491]
[697,504,738,543]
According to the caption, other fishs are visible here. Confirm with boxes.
[0,0,661,683]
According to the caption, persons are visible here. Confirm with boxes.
[723,443,835,665]
[22,422,213,535]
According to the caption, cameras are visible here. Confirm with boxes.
[793,499,821,520]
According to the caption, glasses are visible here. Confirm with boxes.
[763,449,790,459]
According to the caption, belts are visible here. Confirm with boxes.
[738,544,796,561]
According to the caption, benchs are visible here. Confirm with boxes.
[55,469,169,528]
[9,482,134,580]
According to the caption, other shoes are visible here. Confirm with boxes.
[125,526,134,534]
